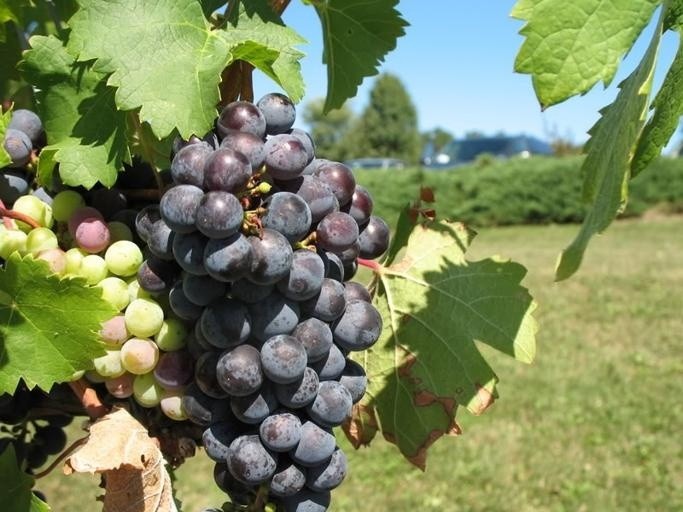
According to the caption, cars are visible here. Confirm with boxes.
[344,158,406,169]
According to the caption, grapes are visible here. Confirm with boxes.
[0,93,391,512]
